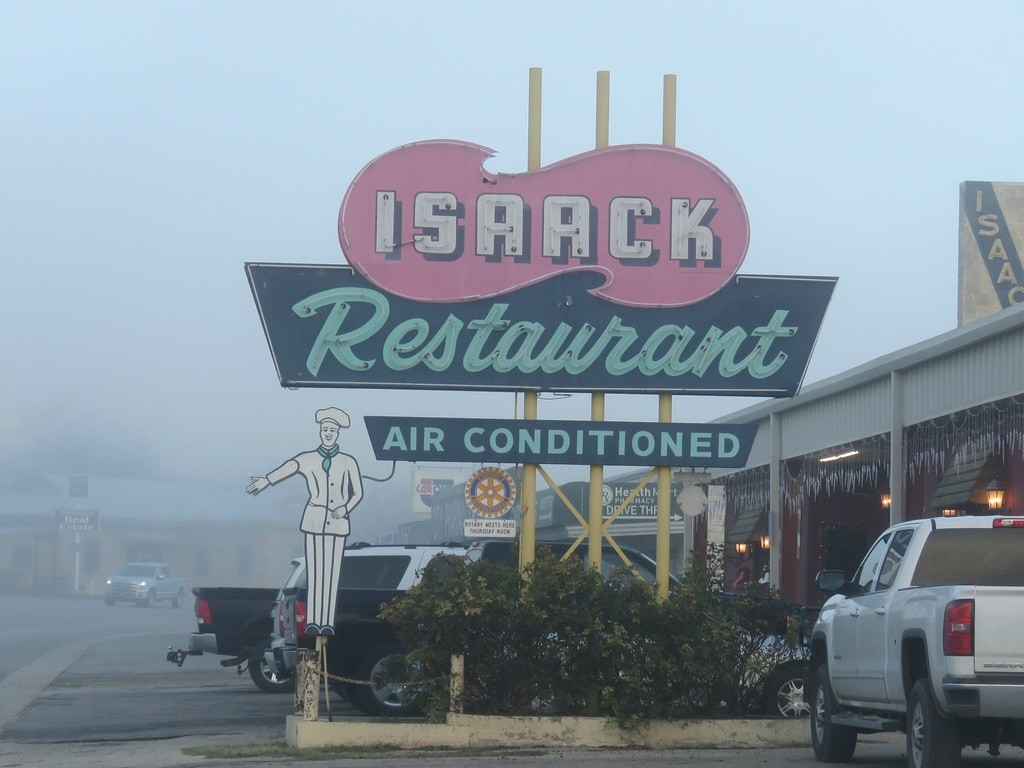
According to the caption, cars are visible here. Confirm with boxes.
[729,625,814,721]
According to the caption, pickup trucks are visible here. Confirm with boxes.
[105,562,188,608]
[808,515,1024,768]
[190,586,297,693]
[278,538,682,718]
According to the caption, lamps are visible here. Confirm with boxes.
[942,505,967,516]
[735,543,752,562]
[760,526,770,557]
[877,484,891,517]
[986,474,1006,516]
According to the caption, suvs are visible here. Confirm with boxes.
[270,542,469,660]
